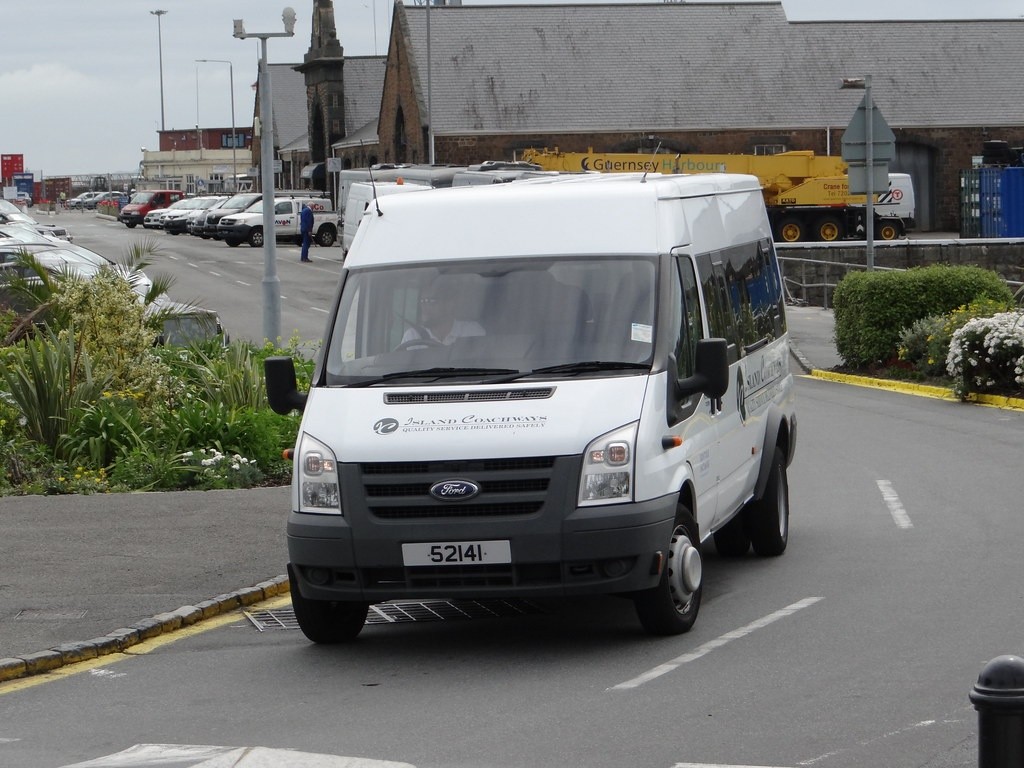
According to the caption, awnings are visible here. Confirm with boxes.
[300,162,326,179]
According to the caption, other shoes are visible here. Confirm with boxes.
[300,258,313,262]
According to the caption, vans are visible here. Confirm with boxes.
[259,170,801,647]
[850,170,916,227]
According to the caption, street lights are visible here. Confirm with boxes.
[230,6,297,354]
[837,73,875,274]
[195,56,238,197]
[149,7,172,130]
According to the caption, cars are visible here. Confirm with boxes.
[0,160,558,364]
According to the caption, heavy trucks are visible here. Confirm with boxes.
[448,147,908,245]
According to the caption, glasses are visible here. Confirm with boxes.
[419,297,439,304]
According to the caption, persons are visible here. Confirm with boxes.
[60,190,66,207]
[400,281,486,351]
[300,202,315,263]
[735,310,773,346]
